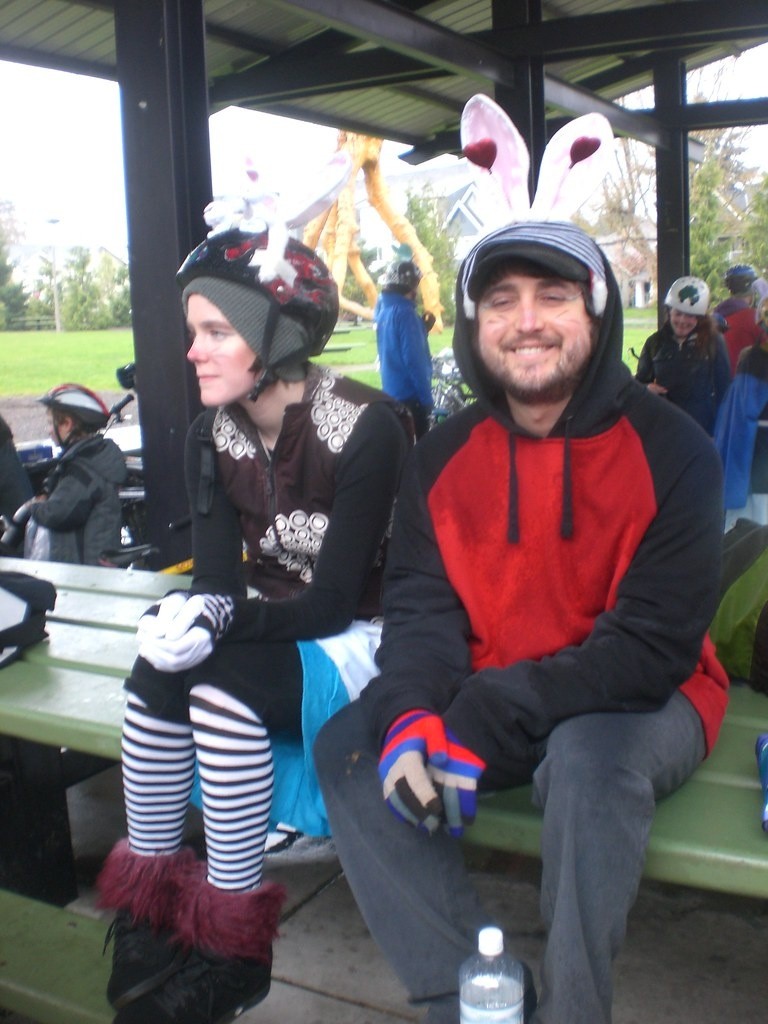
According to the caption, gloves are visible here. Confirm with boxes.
[427,725,487,836]
[135,590,234,673]
[378,709,447,836]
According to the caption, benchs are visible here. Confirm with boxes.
[1,558,768,1024]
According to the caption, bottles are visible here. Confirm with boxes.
[458,926,525,1024]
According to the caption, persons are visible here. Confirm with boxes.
[714,265,767,381]
[372,261,436,444]
[714,277,768,534]
[98,225,416,1023]
[313,222,729,1023]
[634,275,731,438]
[29,383,127,568]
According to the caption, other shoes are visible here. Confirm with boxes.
[521,981,537,1024]
[96,837,197,1012]
[109,875,285,1024]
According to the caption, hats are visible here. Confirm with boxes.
[182,276,318,381]
[468,242,589,302]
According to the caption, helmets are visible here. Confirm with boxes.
[724,266,759,281]
[386,261,422,290]
[35,383,110,427]
[176,222,339,356]
[664,277,711,315]
